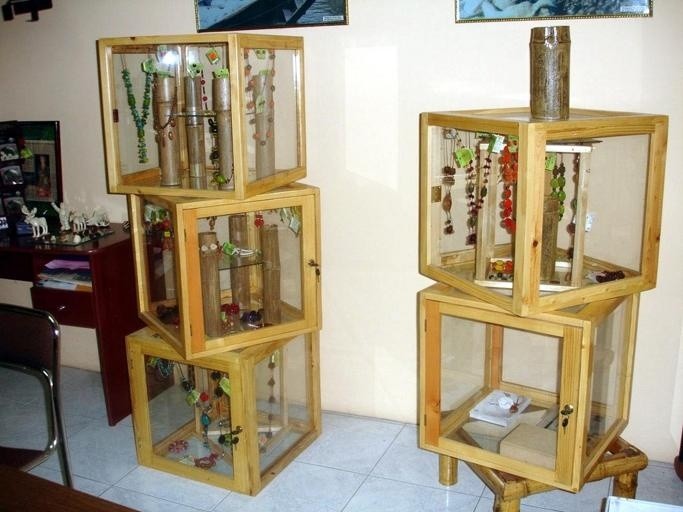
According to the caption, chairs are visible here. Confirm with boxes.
[0,302,75,490]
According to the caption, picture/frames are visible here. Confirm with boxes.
[193,0,349,33]
[454,0,653,24]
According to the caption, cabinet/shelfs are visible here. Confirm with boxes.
[97,32,323,496]
[416,108,668,512]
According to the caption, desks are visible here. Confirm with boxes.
[0,223,175,426]
[0,464,142,512]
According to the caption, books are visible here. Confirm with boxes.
[469,389,532,427]
[37,259,92,293]
